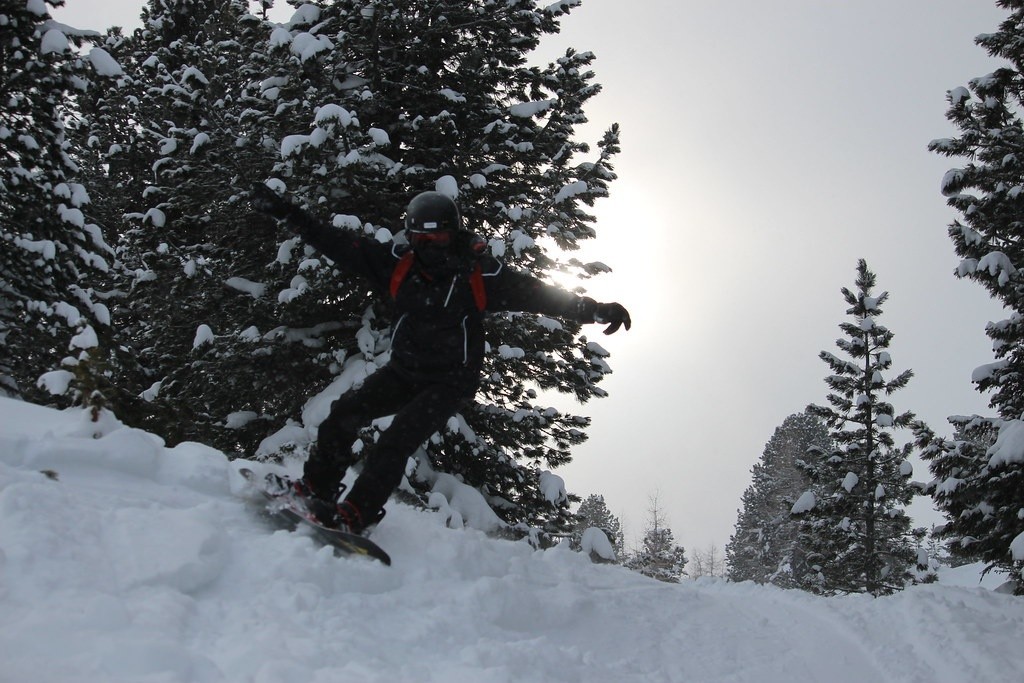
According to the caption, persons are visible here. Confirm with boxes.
[247,180,631,537]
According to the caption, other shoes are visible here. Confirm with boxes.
[298,501,386,540]
[264,473,347,523]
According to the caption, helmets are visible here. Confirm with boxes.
[405,193,460,265]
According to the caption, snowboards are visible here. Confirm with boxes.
[239,466,394,568]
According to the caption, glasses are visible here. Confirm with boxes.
[408,229,455,248]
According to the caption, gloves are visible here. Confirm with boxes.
[251,182,291,220]
[596,303,631,335]
